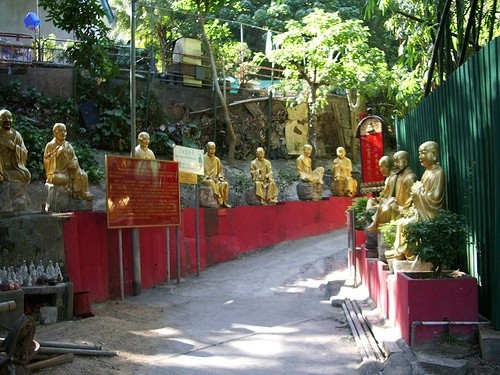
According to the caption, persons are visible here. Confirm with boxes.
[200,141,231,207]
[296,144,325,185]
[44,122,95,202]
[129,132,156,160]
[389,150,418,222]
[331,147,353,197]
[363,155,398,232]
[250,147,279,206]
[384,140,445,260]
[0,258,65,288]
[0,109,33,186]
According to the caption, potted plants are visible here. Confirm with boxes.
[377,218,397,273]
[356,209,377,249]
[395,207,480,346]
